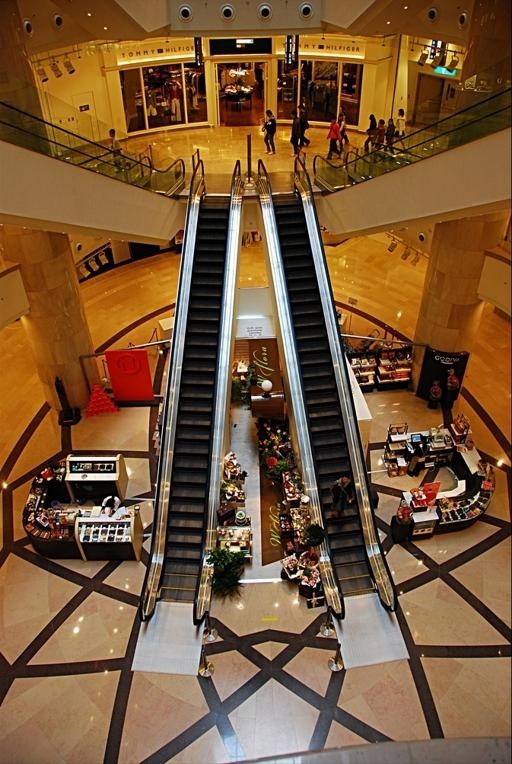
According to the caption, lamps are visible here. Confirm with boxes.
[79,250,109,277]
[387,236,421,267]
[417,44,459,72]
[261,379,273,398]
[35,53,76,82]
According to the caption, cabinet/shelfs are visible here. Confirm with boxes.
[23,452,143,562]
[346,346,413,393]
[382,414,497,544]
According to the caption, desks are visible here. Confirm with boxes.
[216,451,322,598]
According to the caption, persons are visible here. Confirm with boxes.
[446,367,460,391]
[108,128,132,172]
[263,109,276,155]
[337,105,350,152]
[288,110,301,156]
[101,495,122,518]
[407,435,430,477]
[297,103,310,146]
[362,107,407,156]
[325,114,342,159]
[330,475,353,520]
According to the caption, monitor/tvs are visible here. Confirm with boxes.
[411,434,422,442]
